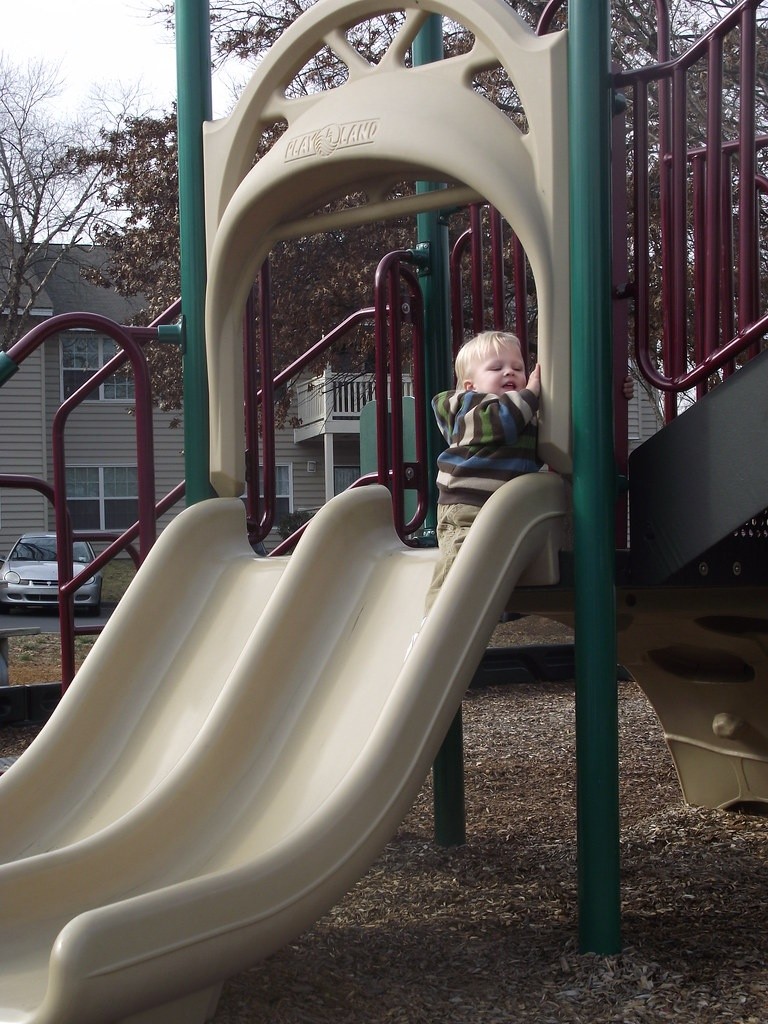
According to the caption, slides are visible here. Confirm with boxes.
[0,473,572,1024]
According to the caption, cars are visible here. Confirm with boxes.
[0,532,104,618]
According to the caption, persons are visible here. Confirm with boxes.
[425,332,635,619]
